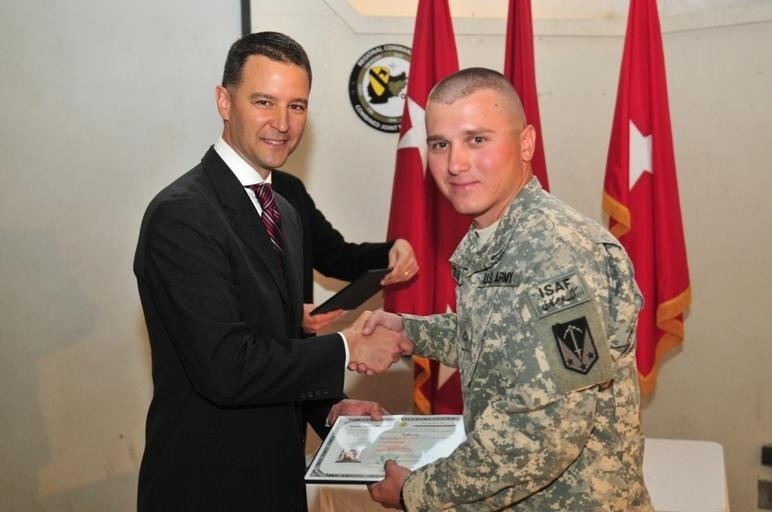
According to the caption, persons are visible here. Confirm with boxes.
[133,29,413,512]
[347,68,652,511]
[273,170,420,334]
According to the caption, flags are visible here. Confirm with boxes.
[601,0,692,402]
[382,0,475,415]
[503,0,548,192]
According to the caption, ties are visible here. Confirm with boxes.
[246,182,283,271]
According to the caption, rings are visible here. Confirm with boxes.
[405,271,409,277]
